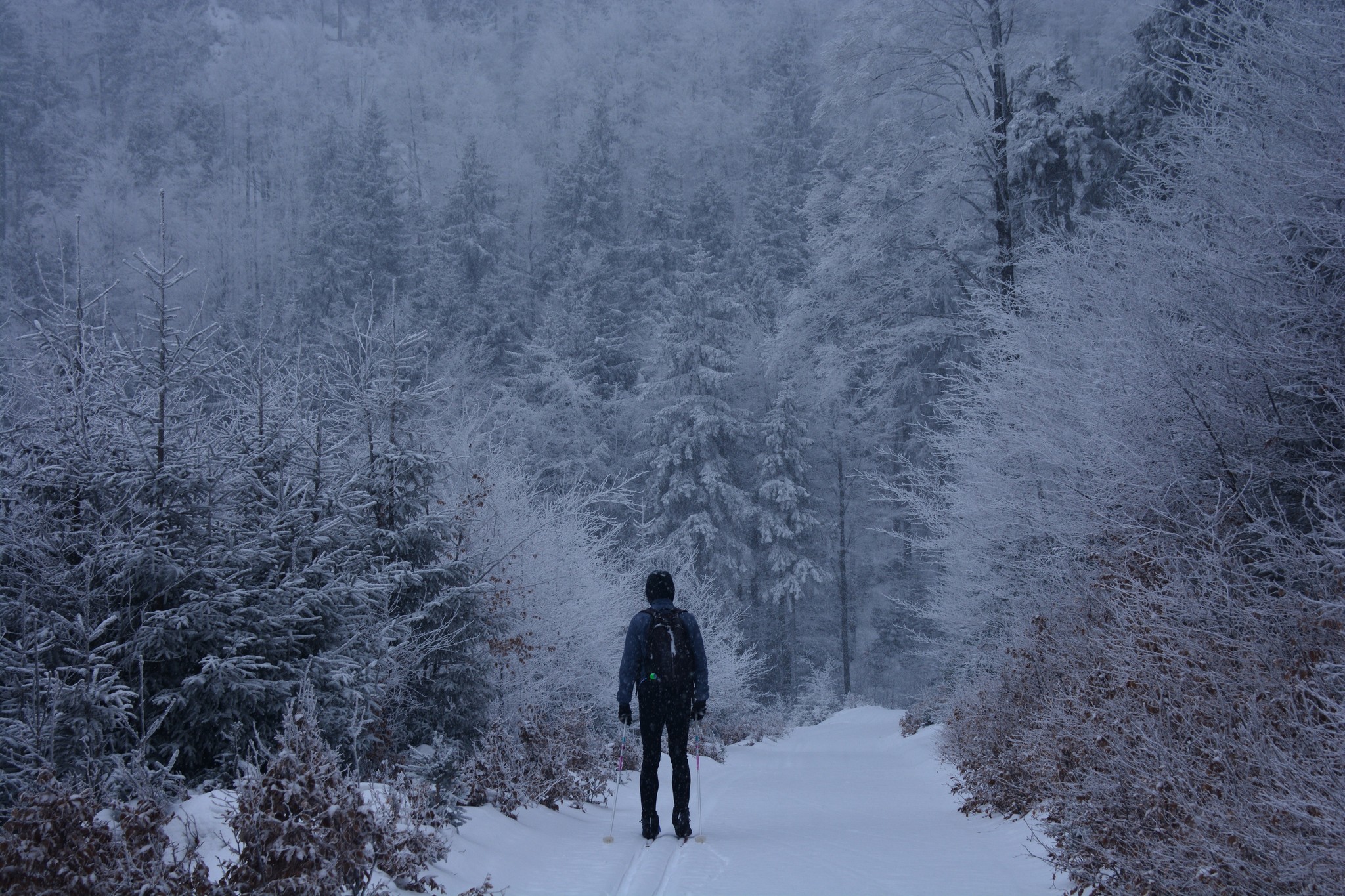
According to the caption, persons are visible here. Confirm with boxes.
[617,571,710,839]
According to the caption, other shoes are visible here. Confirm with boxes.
[672,807,692,838]
[641,810,660,840]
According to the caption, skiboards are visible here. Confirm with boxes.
[645,836,686,848]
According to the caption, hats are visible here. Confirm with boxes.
[645,570,675,606]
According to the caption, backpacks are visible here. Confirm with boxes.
[638,607,692,709]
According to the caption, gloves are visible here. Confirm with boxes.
[690,700,707,721]
[618,704,633,726]
